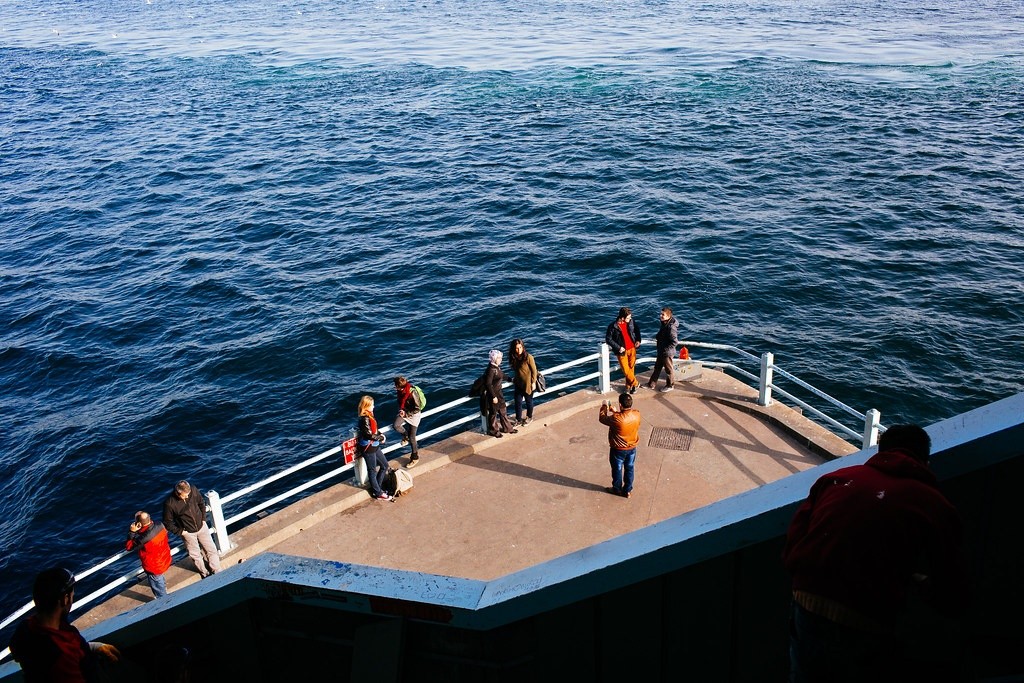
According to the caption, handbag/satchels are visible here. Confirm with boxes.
[535,372,545,392]
[469,365,496,397]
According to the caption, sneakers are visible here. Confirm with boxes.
[523,416,533,426]
[373,490,393,501]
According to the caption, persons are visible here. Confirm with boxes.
[483,350,518,438]
[508,339,537,427]
[640,310,679,393]
[162,480,223,579]
[598,394,640,498]
[357,396,393,501]
[6,568,120,683]
[125,510,172,600]
[392,376,421,468]
[605,307,641,394]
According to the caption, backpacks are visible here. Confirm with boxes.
[409,385,426,410]
[386,467,414,496]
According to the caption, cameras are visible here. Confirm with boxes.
[607,401,611,408]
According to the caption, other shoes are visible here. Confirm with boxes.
[624,389,630,394]
[647,381,656,390]
[606,487,622,496]
[406,458,419,468]
[622,491,630,498]
[489,432,503,438]
[631,383,640,394]
[504,429,518,433]
[401,435,408,445]
[661,384,674,392]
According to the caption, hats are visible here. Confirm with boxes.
[489,350,501,363]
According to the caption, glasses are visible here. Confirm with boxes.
[61,568,75,592]
